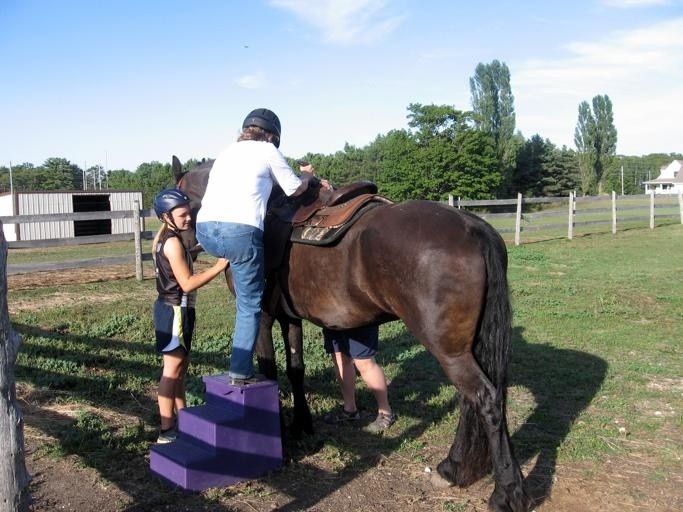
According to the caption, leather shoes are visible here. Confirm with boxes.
[229,373,267,385]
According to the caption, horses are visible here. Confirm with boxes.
[172,155,536,512]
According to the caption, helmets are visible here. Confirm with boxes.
[154,189,193,216]
[242,108,281,137]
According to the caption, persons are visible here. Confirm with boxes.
[318,178,399,429]
[195,109,315,386]
[152,188,228,444]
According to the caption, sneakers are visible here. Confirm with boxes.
[325,404,360,423]
[367,408,398,431]
[157,428,178,444]
